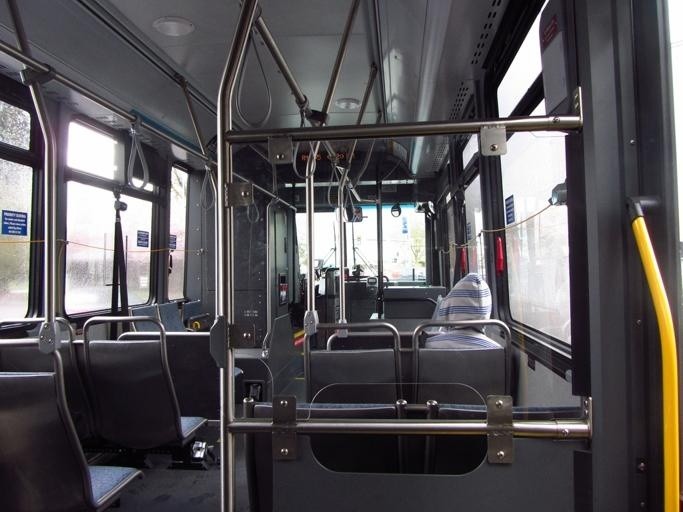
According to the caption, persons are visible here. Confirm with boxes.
[425,272,503,350]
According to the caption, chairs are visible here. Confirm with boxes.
[81,315,209,469]
[1,336,145,511]
[302,324,515,471]
[1,317,97,454]
[128,297,213,333]
